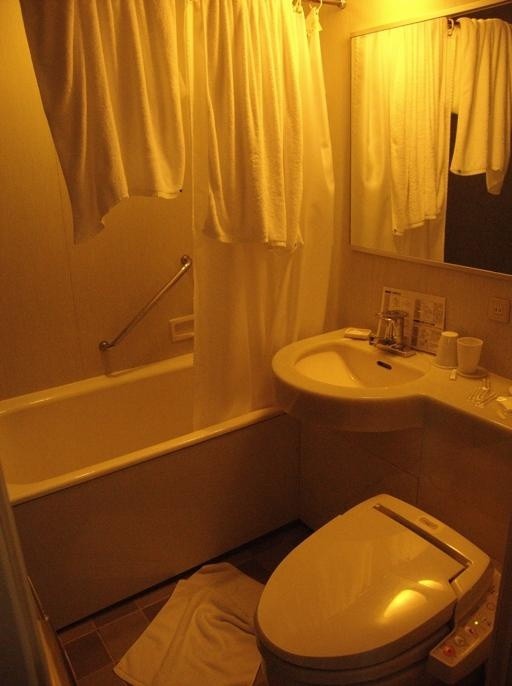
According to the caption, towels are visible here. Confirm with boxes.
[112,561,266,685]
[384,17,511,235]
[17,0,191,246]
[15,2,311,248]
[194,0,311,257]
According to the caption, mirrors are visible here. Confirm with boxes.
[348,0,511,279]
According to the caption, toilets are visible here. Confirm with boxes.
[254,491,497,685]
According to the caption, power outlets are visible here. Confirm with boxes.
[486,296,511,323]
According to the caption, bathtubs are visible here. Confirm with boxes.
[1,350,283,504]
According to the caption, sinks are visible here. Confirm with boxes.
[271,325,427,431]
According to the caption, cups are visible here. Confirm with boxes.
[435,331,484,375]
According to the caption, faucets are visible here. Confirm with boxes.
[370,307,415,357]
